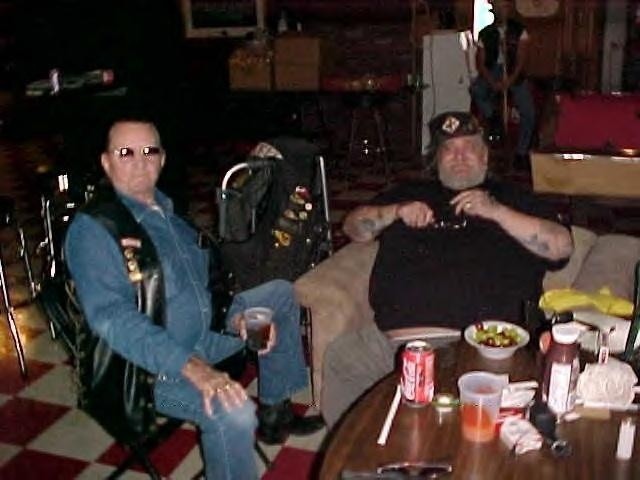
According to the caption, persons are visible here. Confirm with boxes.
[470,1,536,169]
[62,116,325,480]
[343,110,573,333]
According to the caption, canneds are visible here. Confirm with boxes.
[399,340,434,408]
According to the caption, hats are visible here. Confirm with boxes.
[426,110,483,148]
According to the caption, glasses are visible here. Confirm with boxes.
[107,143,164,159]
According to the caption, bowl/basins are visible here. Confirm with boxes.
[464,320,531,360]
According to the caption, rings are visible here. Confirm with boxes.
[465,201,471,208]
[223,383,231,391]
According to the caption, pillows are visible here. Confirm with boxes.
[554,92,640,151]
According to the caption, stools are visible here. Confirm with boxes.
[340,91,395,186]
[0,194,58,377]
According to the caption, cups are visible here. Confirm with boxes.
[244,305,276,353]
[456,371,504,445]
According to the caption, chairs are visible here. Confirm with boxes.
[293,224,640,434]
[39,271,277,480]
[218,161,280,237]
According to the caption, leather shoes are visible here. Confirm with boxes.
[257,414,327,445]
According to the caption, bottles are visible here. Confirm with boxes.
[541,323,580,424]
[614,417,635,463]
